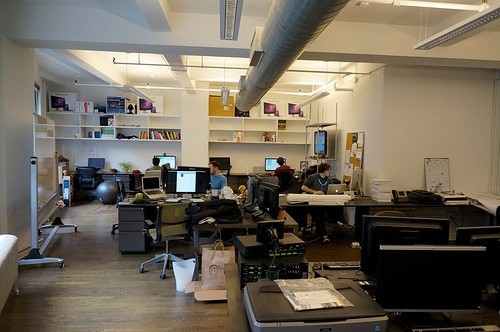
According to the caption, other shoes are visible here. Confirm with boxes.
[322,235,330,244]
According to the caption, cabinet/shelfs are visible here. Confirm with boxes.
[117,207,145,255]
[98,172,141,189]
[47,111,181,141]
[210,116,311,145]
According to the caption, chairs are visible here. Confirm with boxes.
[303,208,339,239]
[140,201,193,278]
[111,180,142,235]
[145,170,166,192]
[275,168,294,192]
[76,166,97,200]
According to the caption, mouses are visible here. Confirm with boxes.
[313,262,322,270]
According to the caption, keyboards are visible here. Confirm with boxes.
[412,325,500,332]
[323,262,360,270]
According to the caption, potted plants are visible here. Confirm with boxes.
[119,161,131,172]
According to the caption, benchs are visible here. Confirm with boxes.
[0,234,21,313]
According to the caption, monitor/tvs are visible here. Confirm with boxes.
[314,130,327,156]
[139,98,153,110]
[289,104,301,114]
[256,180,279,220]
[51,96,65,108]
[209,157,230,170]
[360,215,500,328]
[156,156,211,195]
[265,158,286,171]
[88,158,105,169]
[264,102,276,114]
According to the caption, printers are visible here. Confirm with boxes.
[243,278,389,332]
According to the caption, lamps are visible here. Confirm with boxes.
[222,57,230,106]
[295,63,329,107]
[123,54,155,103]
[414,5,500,49]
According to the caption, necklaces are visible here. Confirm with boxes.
[318,178,328,186]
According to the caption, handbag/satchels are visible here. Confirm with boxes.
[184,199,242,229]
[408,190,442,204]
[172,258,196,291]
[201,239,235,290]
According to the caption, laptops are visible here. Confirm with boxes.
[142,176,168,200]
[327,184,347,195]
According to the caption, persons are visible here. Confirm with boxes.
[147,157,163,186]
[301,162,347,244]
[209,161,227,194]
[269,157,290,176]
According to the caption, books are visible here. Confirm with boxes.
[139,130,148,139]
[89,128,114,138]
[149,130,181,140]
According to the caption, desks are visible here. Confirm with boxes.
[117,200,158,207]
[224,263,500,332]
[192,208,299,252]
[220,173,252,176]
[279,194,493,243]
